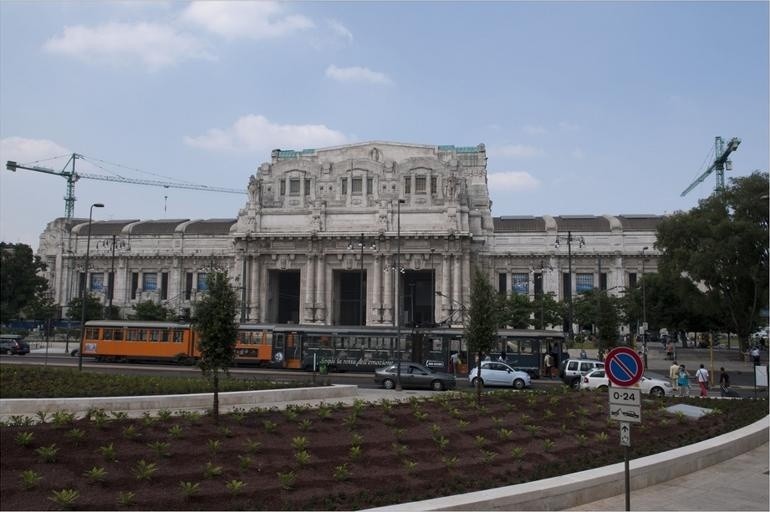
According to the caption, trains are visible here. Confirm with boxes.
[81,319,570,379]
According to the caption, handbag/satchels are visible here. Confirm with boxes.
[704,383,708,388]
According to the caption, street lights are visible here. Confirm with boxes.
[396,199,405,390]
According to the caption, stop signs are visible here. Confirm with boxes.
[604,347,644,386]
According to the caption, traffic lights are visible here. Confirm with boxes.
[642,246,648,377]
[78,203,104,371]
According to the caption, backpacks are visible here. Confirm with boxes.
[548,356,554,364]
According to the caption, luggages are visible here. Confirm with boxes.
[722,385,738,397]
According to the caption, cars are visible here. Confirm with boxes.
[374,361,456,391]
[468,361,532,390]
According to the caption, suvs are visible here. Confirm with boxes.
[0,335,30,355]
[558,359,673,396]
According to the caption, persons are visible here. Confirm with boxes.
[485,353,492,369]
[719,367,730,397]
[543,352,554,379]
[664,342,674,360]
[669,360,680,391]
[677,364,690,396]
[497,351,509,369]
[694,364,710,396]
[450,348,461,379]
[751,346,761,374]
